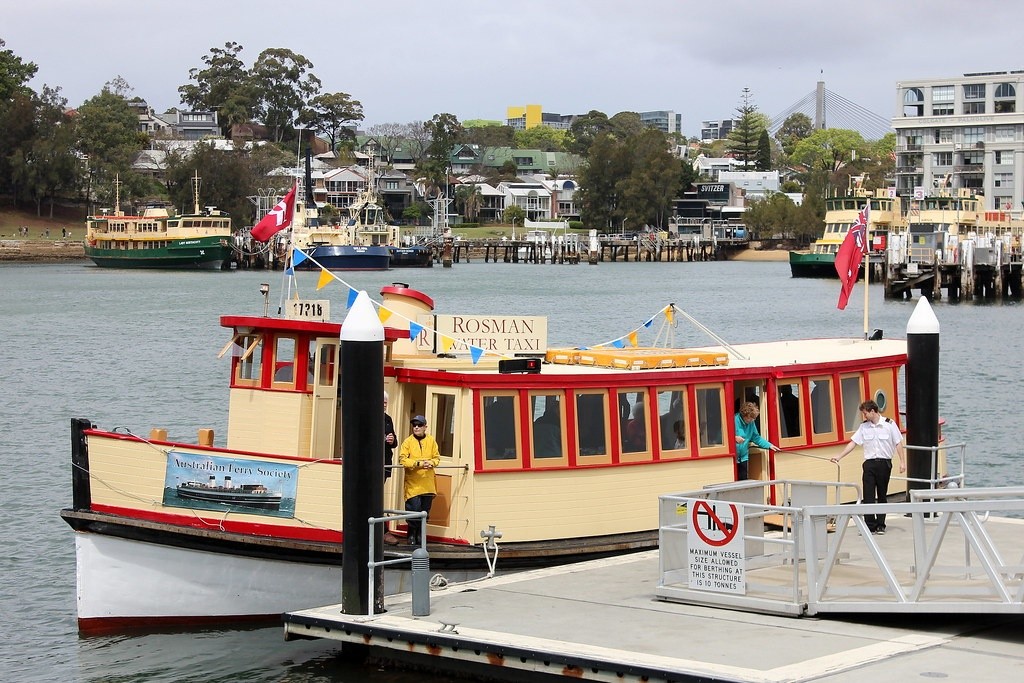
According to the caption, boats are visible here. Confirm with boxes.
[273,132,435,272]
[174,473,282,511]
[84,168,235,272]
[787,172,1024,279]
[61,125,953,642]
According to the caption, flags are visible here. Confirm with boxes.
[250,184,296,243]
[834,204,870,310]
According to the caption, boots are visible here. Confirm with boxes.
[416,525,421,545]
[407,524,416,545]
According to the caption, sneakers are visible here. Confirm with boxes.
[384,532,398,546]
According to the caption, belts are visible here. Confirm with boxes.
[866,458,891,462]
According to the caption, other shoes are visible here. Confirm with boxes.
[871,528,877,535]
[878,526,884,535]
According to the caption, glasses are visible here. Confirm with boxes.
[413,423,424,427]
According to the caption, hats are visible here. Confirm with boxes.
[410,415,426,425]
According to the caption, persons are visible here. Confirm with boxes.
[68,231,72,238]
[18,225,23,236]
[398,415,441,546]
[384,390,398,545]
[45,227,49,238]
[830,399,906,535]
[532,399,563,458]
[780,384,799,437]
[22,226,29,237]
[628,398,682,451]
[62,227,67,238]
[274,349,313,384]
[734,401,781,480]
[673,418,685,448]
